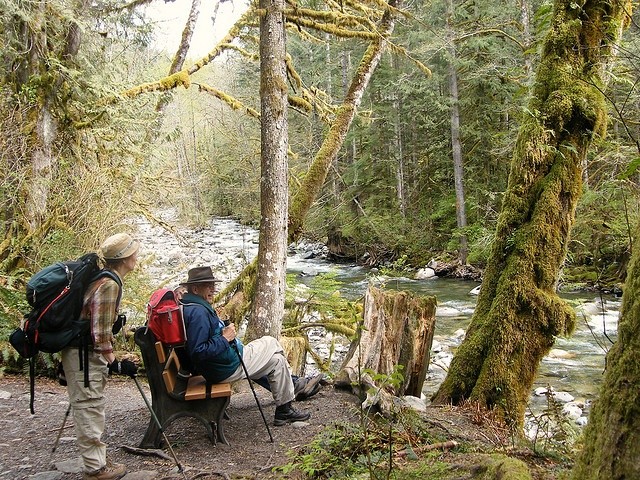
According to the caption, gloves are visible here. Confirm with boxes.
[107,358,138,379]
[112,315,126,334]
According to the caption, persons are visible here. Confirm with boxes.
[166,284,322,401]
[178,267,324,427]
[60,232,142,480]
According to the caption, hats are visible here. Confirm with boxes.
[100,232,139,260]
[179,266,223,285]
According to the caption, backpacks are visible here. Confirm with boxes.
[9,252,121,415]
[144,287,202,348]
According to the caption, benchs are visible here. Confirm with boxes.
[134,322,233,446]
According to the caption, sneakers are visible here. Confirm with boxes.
[302,373,324,398]
[273,405,310,426]
[85,456,126,480]
